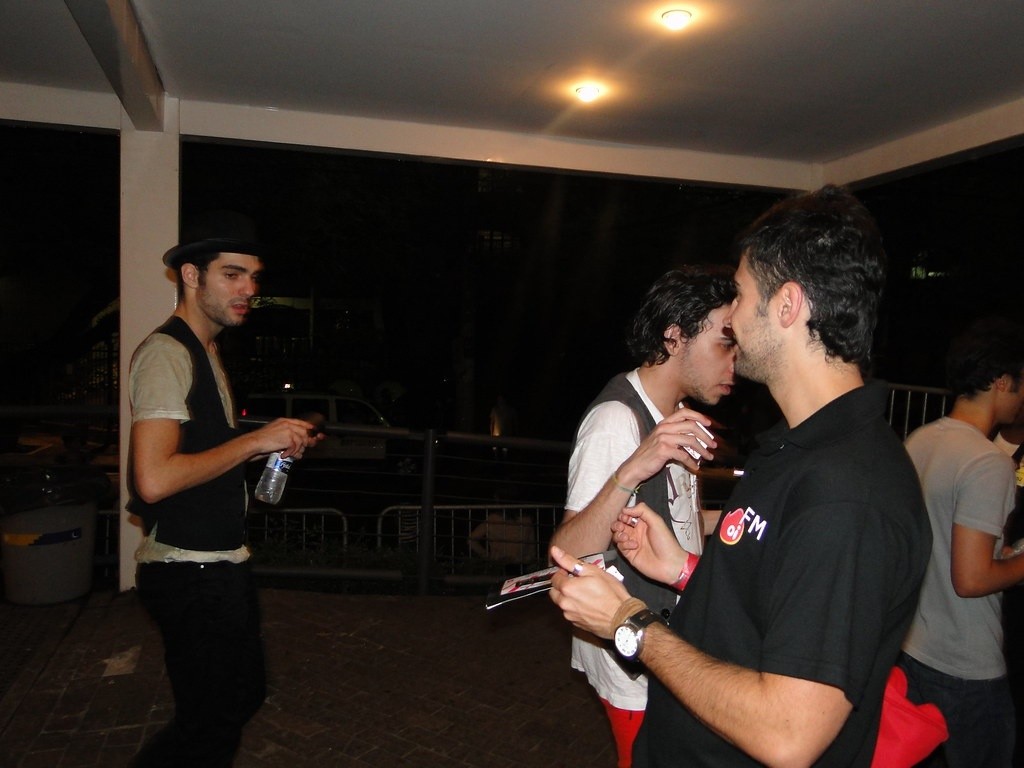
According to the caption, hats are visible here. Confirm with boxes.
[162,209,281,271]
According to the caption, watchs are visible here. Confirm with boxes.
[613,609,671,675]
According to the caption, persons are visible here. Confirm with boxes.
[127,194,327,768]
[470,491,536,597]
[551,258,738,767]
[552,182,933,768]
[903,337,1024,768]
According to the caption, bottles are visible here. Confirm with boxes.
[254,450,294,504]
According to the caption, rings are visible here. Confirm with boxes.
[629,517,638,527]
[572,560,584,577]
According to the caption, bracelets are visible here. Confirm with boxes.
[611,597,648,637]
[612,472,634,494]
